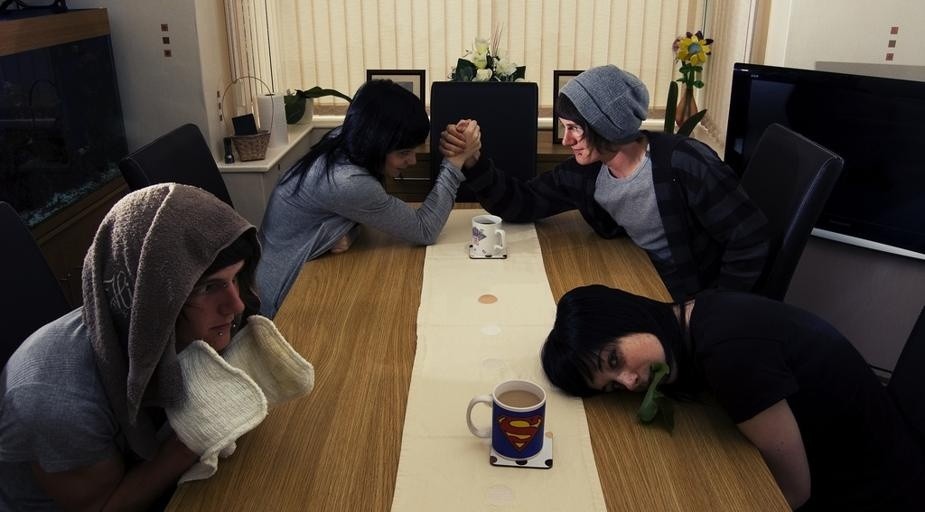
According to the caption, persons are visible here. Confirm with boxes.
[438,62,781,301]
[543,284,925,512]
[0,178,261,512]
[251,76,484,323]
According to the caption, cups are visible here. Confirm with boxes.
[465,378,548,459]
[470,214,506,257]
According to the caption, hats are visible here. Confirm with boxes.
[559,63,650,146]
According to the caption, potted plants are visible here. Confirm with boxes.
[282,84,356,125]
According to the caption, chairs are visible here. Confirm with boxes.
[1,202,65,373]
[430,81,538,202]
[877,298,925,512]
[117,124,236,217]
[739,123,843,313]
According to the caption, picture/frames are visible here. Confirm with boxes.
[552,69,598,143]
[365,68,426,114]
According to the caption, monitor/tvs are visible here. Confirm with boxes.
[725,62,925,261]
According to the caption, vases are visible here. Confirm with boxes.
[674,90,698,129]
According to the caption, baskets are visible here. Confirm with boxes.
[221,76,275,162]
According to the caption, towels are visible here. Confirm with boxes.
[80,183,264,468]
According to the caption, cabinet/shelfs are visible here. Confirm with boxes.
[214,121,312,228]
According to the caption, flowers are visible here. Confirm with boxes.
[669,29,716,124]
[440,20,523,83]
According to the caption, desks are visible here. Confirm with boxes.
[391,127,590,202]
[167,189,786,512]
